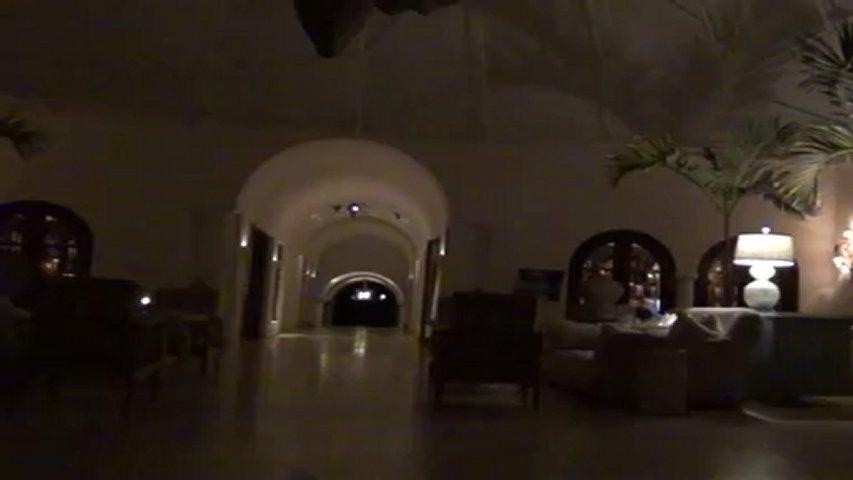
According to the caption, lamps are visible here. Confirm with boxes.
[732,226,795,312]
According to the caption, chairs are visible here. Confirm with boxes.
[430,291,546,412]
[19,276,138,433]
